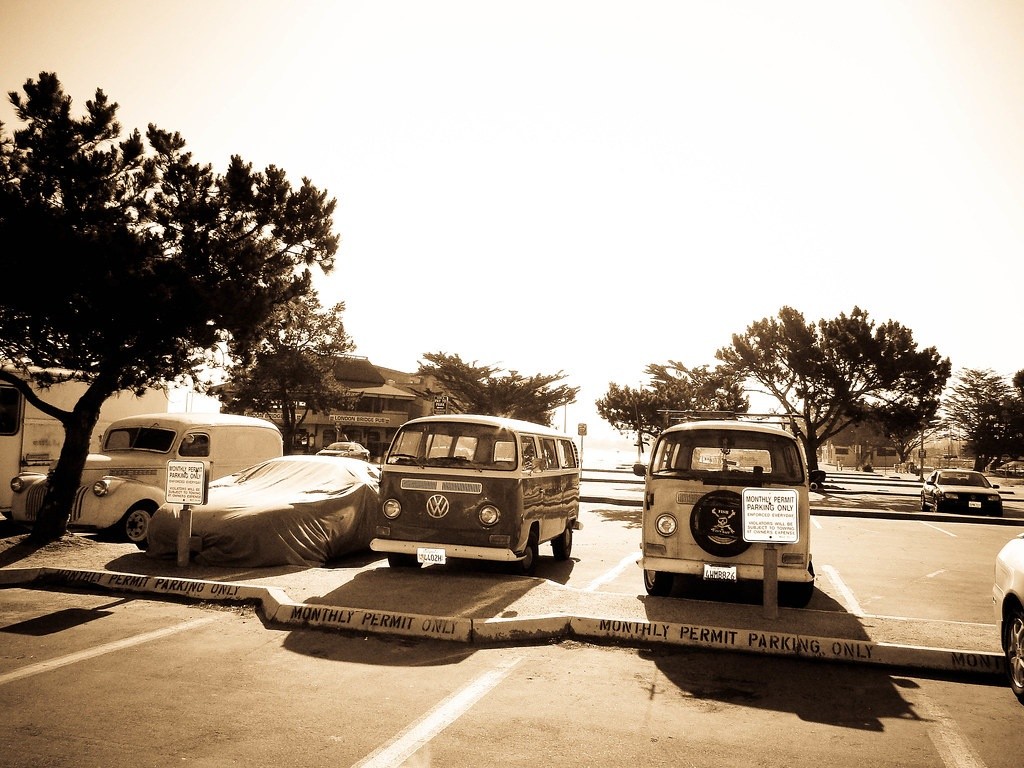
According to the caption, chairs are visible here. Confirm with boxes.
[940,478,951,485]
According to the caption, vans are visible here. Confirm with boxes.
[633,419,827,603]
[9,413,284,543]
[369,412,583,575]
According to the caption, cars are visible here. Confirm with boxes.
[919,467,1005,517]
[425,445,473,460]
[316,442,371,463]
[992,530,1024,702]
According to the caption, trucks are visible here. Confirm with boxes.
[0,362,170,523]
